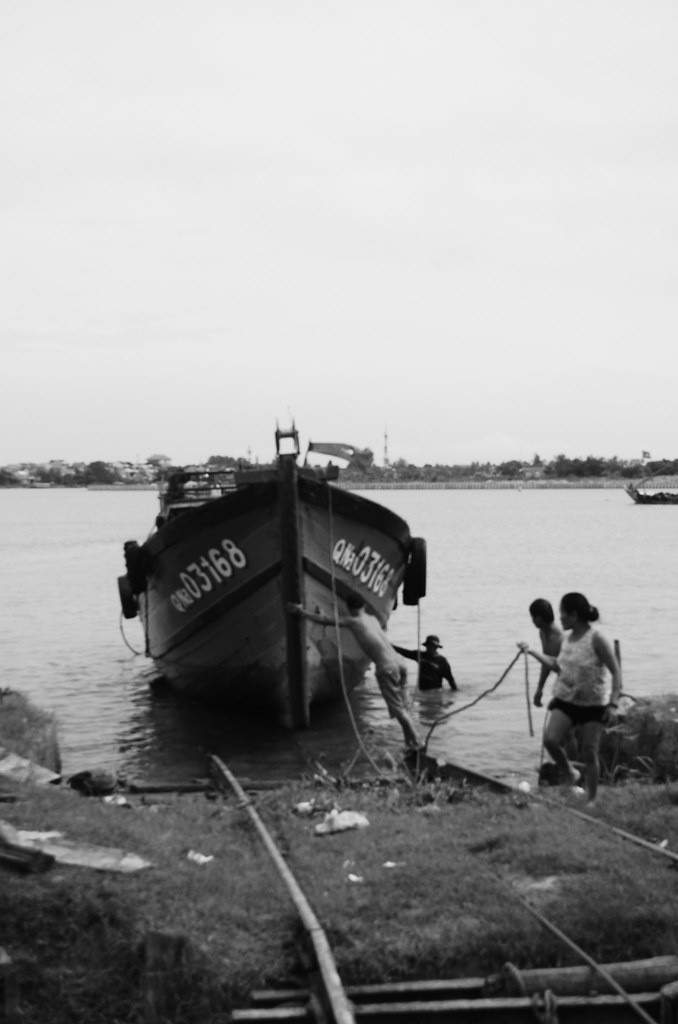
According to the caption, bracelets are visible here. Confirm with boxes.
[609,703,618,708]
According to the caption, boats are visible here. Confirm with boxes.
[156,467,238,524]
[117,421,430,729]
[616,483,676,508]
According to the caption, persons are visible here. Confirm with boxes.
[392,635,457,691]
[290,593,425,753]
[516,592,623,808]
[529,599,577,761]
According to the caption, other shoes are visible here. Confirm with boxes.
[558,767,581,796]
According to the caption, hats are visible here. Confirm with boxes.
[422,635,443,648]
